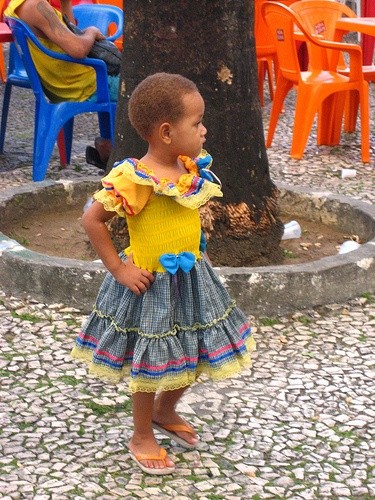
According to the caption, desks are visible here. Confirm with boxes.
[330,17,375,71]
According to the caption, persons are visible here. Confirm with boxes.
[71,72,257,475]
[3,0,121,102]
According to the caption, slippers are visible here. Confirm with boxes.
[152,420,201,449]
[86,146,111,171]
[130,448,176,475]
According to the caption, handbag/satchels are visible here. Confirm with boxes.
[63,14,123,77]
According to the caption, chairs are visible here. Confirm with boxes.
[0,0,123,182]
[254,0,370,162]
